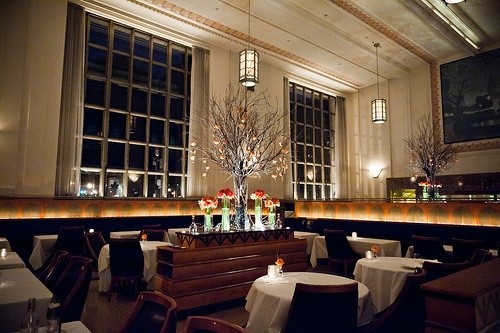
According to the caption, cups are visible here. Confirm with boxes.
[365,251,373,262]
[352,232,357,240]
[1,248,8,258]
[267,265,280,281]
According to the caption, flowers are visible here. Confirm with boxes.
[197,187,281,224]
[401,112,458,175]
[275,258,284,267]
[371,245,378,253]
[186,84,302,182]
[419,181,429,186]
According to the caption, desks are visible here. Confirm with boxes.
[293,231,320,254]
[167,228,190,245]
[245,271,373,333]
[0,235,91,333]
[96,238,174,291]
[310,235,401,267]
[406,246,498,258]
[353,254,442,317]
[110,231,141,239]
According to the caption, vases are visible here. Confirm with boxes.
[204,214,213,231]
[255,207,264,230]
[427,174,435,199]
[221,208,230,229]
[234,179,247,230]
[268,213,276,229]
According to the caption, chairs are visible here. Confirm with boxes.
[31,229,500,333]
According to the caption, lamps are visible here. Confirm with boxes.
[239,0,259,87]
[371,44,387,123]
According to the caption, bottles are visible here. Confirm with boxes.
[275,213,282,230]
[189,218,198,234]
